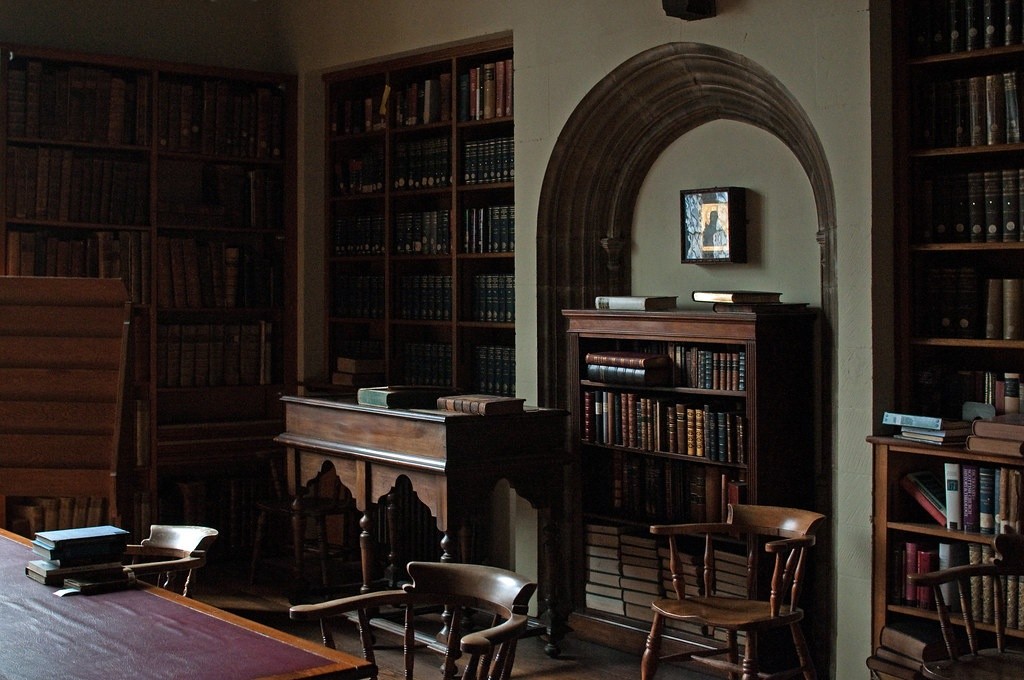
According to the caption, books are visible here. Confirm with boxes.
[393,136,452,191]
[357,384,449,409]
[331,270,514,396]
[6,229,151,304]
[331,354,385,401]
[330,142,384,196]
[162,459,291,587]
[157,156,283,227]
[461,205,514,255]
[865,0,1024,679]
[7,142,150,227]
[25,524,130,586]
[392,209,452,256]
[395,70,452,127]
[10,498,105,539]
[335,213,386,257]
[456,59,513,123]
[158,76,286,160]
[306,477,443,563]
[463,136,514,185]
[157,319,283,389]
[118,315,150,467]
[330,92,386,135]
[117,487,152,564]
[7,60,152,146]
[156,236,283,308]
[584,342,749,647]
[437,394,527,417]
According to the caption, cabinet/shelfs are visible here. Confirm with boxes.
[321,33,516,408]
[865,0,1024,422]
[0,41,302,573]
[869,430,1024,680]
[558,306,817,680]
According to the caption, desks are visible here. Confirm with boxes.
[265,387,573,660]
[0,526,382,680]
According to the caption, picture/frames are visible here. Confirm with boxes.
[675,186,747,264]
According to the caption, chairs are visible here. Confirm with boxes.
[903,559,1024,680]
[285,548,541,680]
[638,502,831,680]
[245,445,353,601]
[122,521,222,599]
